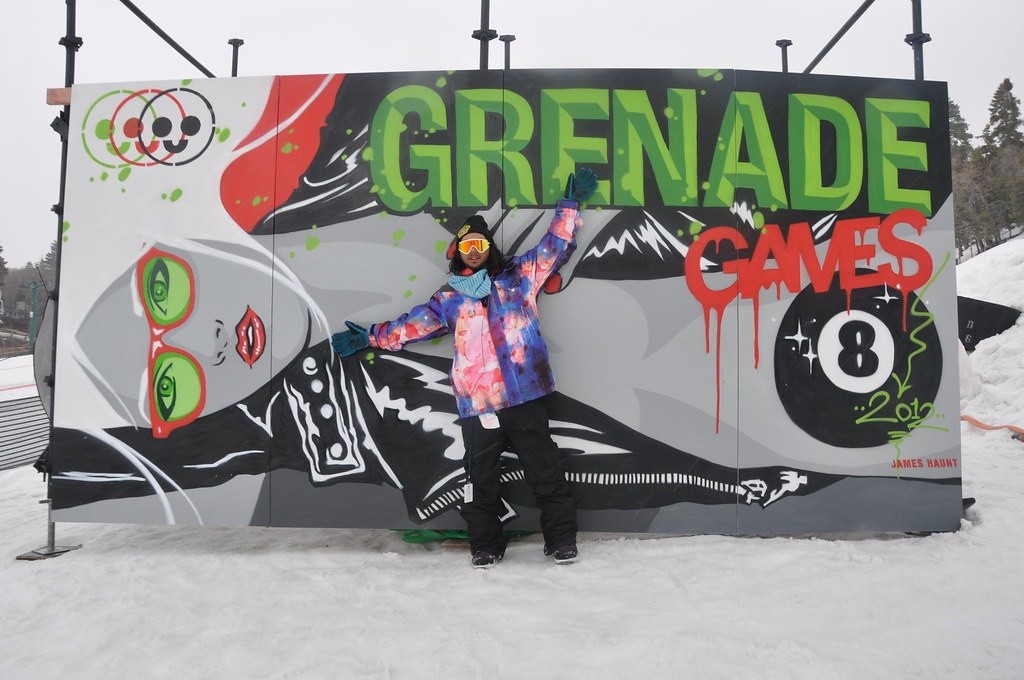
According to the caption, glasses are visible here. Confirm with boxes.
[458,239,490,254]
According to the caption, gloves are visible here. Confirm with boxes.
[331,321,370,358]
[564,167,598,203]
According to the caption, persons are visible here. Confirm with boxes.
[330,166,599,569]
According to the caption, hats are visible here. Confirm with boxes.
[456,215,494,247]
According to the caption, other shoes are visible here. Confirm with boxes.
[472,549,505,569]
[553,544,579,564]
